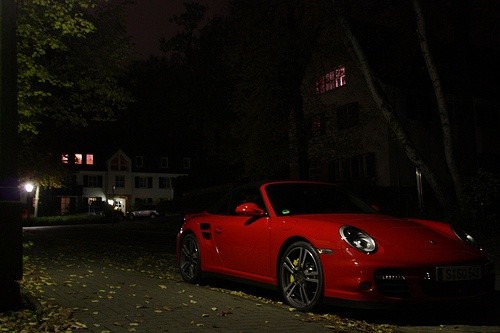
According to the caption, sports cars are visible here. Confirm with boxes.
[176,178,497,314]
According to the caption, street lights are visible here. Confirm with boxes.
[24,181,40,217]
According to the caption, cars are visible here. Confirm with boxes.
[126,205,161,220]
